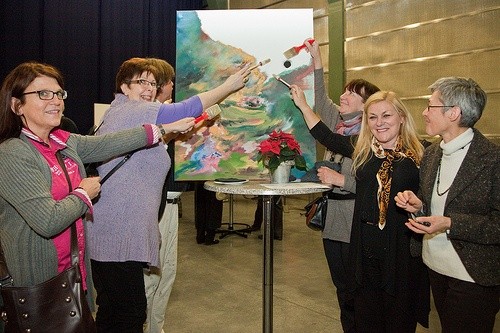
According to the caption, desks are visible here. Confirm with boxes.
[204,175,335,333]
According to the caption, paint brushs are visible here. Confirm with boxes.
[273,73,291,87]
[250,58,270,70]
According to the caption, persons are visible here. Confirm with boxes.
[289,38,500,333]
[0,62,196,333]
[85,57,251,333]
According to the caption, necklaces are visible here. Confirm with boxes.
[436,159,450,196]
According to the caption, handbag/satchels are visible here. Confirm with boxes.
[0,262,95,333]
[304,195,328,232]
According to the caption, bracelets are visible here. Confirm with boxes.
[156,123,165,136]
[74,186,83,190]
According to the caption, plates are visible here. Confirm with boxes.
[214,179,249,185]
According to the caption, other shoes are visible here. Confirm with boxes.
[198,237,219,245]
[258,233,283,240]
[247,226,260,232]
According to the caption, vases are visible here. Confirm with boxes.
[263,160,295,184]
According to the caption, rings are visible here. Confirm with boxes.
[244,78,247,82]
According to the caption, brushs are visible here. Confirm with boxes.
[193,104,222,125]
[283,39,315,60]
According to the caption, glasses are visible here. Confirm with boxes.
[19,88,68,100]
[122,79,160,90]
[427,104,454,112]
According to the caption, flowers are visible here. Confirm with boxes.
[250,129,310,173]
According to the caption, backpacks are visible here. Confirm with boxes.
[78,122,133,204]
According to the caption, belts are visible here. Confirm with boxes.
[166,198,178,204]
